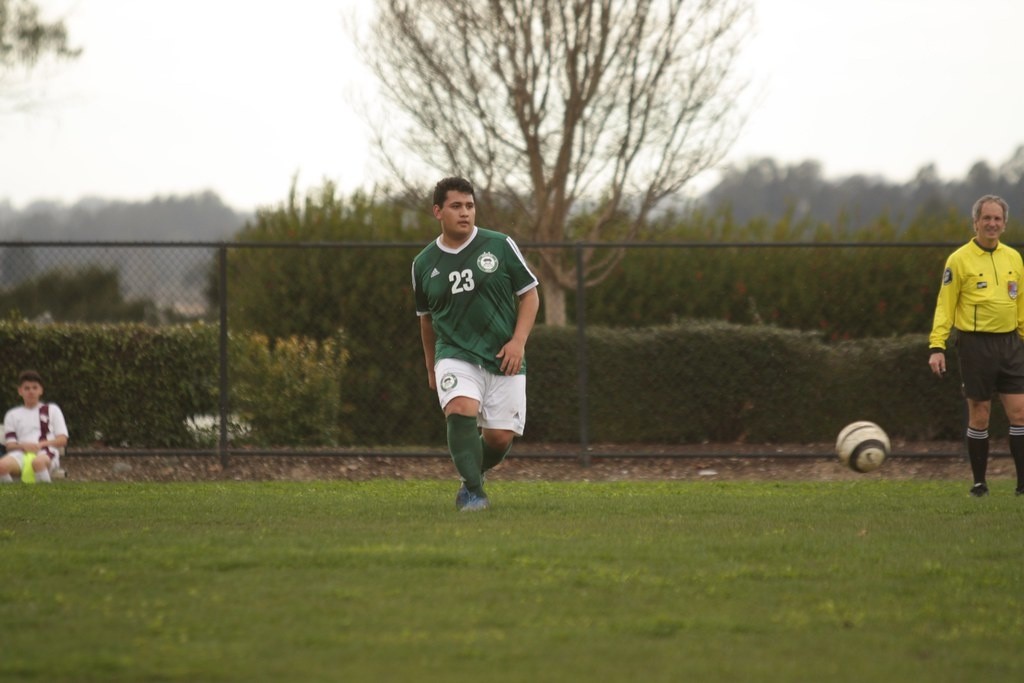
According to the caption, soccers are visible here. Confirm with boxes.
[835,420,892,473]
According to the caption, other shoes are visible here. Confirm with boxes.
[461,494,489,512]
[1015,486,1024,496]
[455,471,486,510]
[970,484,988,498]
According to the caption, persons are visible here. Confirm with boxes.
[929,194,1024,496]
[0,372,68,483]
[411,177,539,511]
